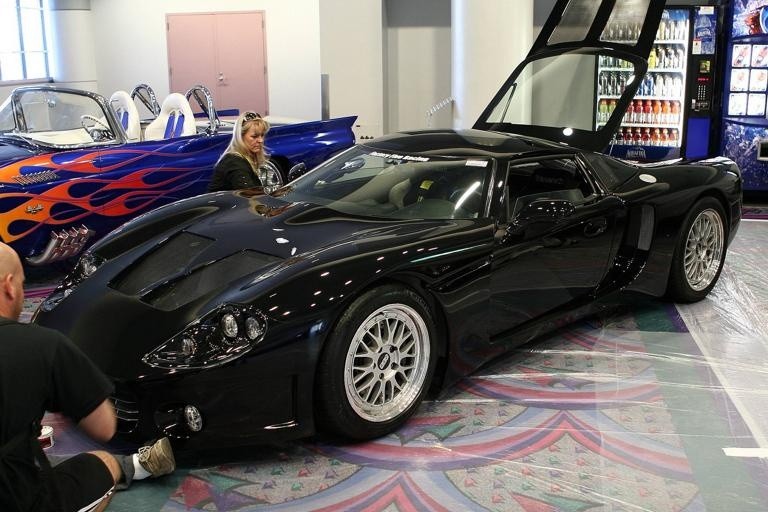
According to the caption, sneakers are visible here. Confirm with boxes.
[136,436,176,476]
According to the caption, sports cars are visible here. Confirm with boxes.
[31,0,745,451]
[0,86,357,267]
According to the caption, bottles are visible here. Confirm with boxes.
[693,9,714,54]
[734,46,749,66]
[631,17,685,69]
[599,99,680,124]
[616,127,679,147]
[754,46,768,65]
[601,73,681,97]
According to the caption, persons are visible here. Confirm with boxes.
[204,109,306,193]
[212,187,296,221]
[43,435,178,491]
[0,239,125,512]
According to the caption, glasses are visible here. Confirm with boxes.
[241,112,262,127]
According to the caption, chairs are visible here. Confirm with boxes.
[385,168,526,229]
[93,91,201,143]
